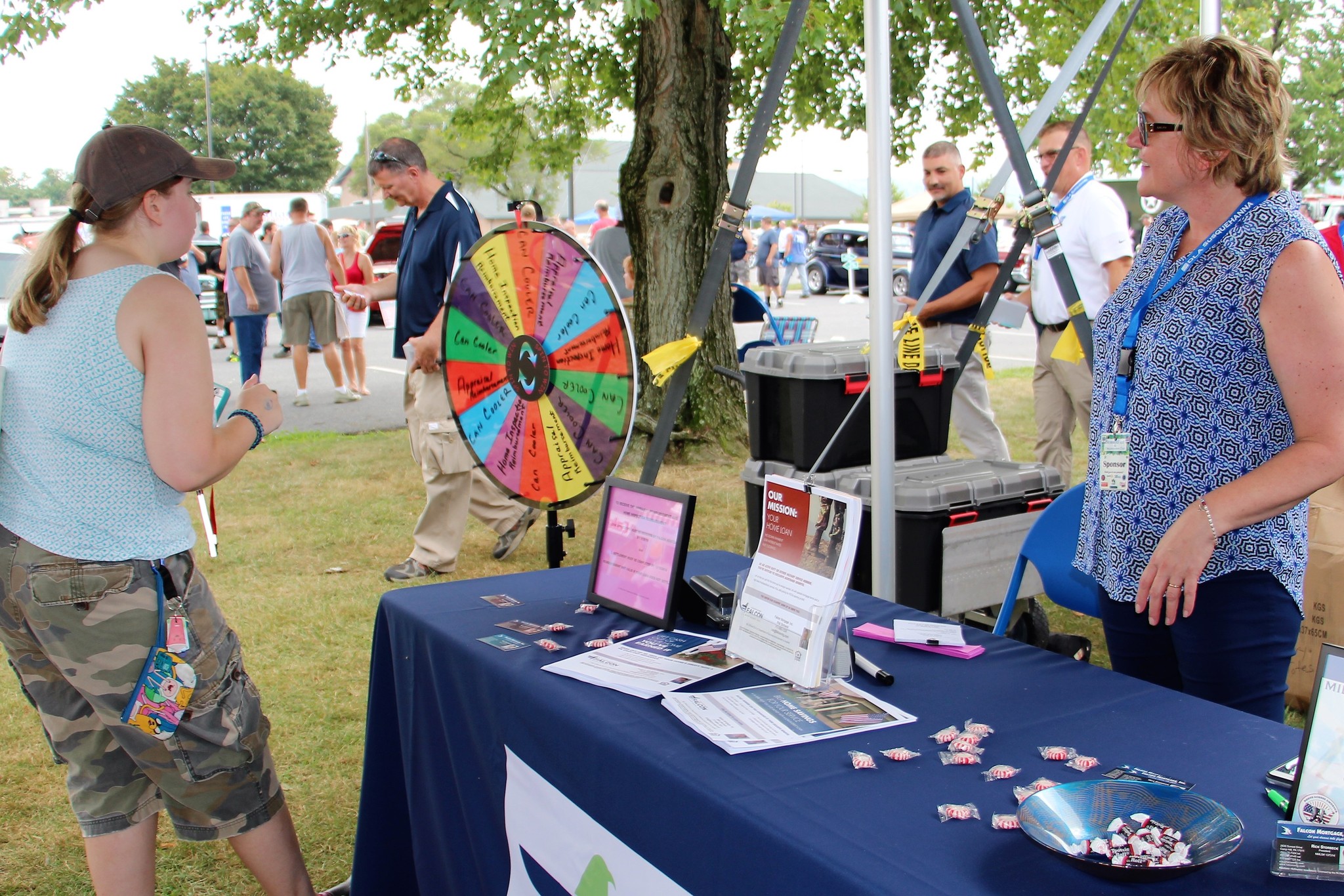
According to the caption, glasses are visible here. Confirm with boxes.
[370,147,410,168]
[1034,146,1080,164]
[1136,103,1186,146]
[336,233,351,239]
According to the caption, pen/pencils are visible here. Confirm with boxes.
[854,651,895,687]
[197,490,218,559]
[1264,788,1289,814]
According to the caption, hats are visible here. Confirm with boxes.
[70,122,237,225]
[241,202,271,217]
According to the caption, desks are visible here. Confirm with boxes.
[353,549,1344,896]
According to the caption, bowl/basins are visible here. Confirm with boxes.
[1017,779,1245,872]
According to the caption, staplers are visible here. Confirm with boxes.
[689,575,736,631]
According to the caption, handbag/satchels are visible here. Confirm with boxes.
[118,646,196,741]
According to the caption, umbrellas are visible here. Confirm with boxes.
[742,204,796,222]
[566,205,616,225]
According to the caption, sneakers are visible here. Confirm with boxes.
[292,391,309,406]
[383,556,439,583]
[491,504,544,562]
[331,388,361,403]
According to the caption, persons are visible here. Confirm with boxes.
[617,255,635,303]
[773,219,810,260]
[746,216,783,309]
[157,215,388,362]
[335,137,550,584]
[0,121,330,896]
[781,219,811,299]
[586,201,636,298]
[521,204,536,221]
[729,226,754,292]
[545,217,562,229]
[269,197,363,408]
[563,221,575,237]
[896,140,1012,464]
[585,200,618,244]
[1006,120,1136,496]
[1072,37,1344,727]
[226,201,281,390]
[326,225,375,397]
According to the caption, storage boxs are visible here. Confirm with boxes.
[715,337,1065,615]
[1285,474,1344,711]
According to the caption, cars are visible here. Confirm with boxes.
[797,198,1034,298]
[361,222,404,283]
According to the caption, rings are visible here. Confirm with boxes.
[1167,583,1182,588]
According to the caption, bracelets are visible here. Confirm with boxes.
[1198,496,1219,546]
[227,409,264,450]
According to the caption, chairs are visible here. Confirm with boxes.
[729,283,819,363]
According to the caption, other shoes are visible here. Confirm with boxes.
[213,336,226,349]
[225,350,240,362]
[272,347,292,358]
